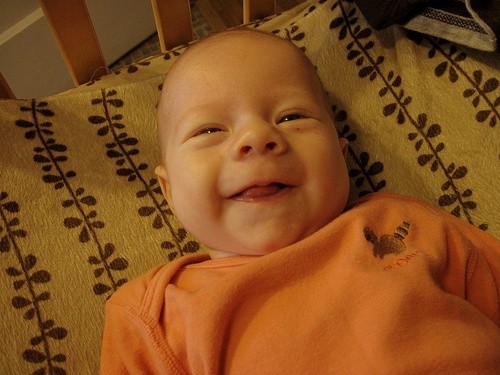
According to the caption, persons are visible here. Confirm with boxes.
[100,27,499,375]
